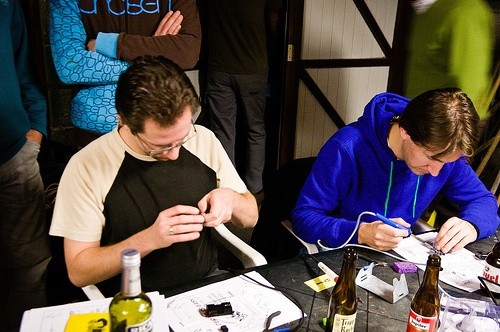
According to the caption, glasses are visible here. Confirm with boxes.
[118,111,197,157]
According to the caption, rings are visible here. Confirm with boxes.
[170,226,174,234]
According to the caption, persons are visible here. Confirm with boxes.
[294,86,500,257]
[49,56,258,298]
[0,0,56,308]
[197,1,279,205]
[405,0,492,144]
[50,0,203,155]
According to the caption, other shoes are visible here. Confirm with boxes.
[252,189,264,213]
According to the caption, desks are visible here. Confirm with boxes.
[12,220,500,331]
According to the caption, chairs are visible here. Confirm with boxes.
[280,214,438,254]
[63,220,272,301]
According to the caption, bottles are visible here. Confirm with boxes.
[480,241,500,299]
[325,248,358,332]
[407,254,440,332]
[108,248,153,332]
